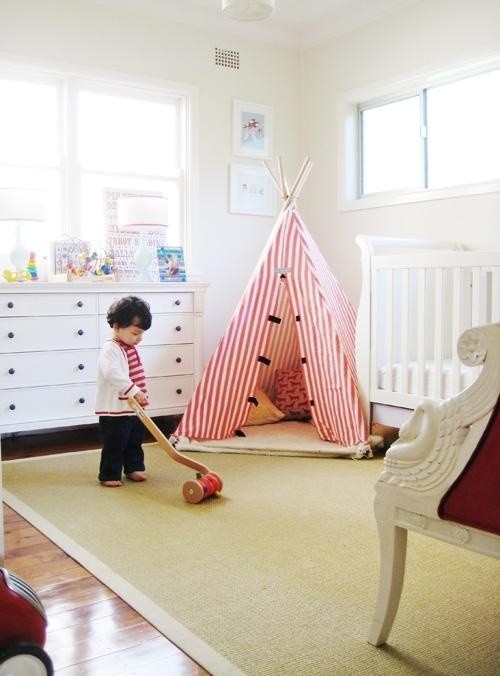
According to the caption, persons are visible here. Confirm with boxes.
[168,253,179,276]
[91,293,155,486]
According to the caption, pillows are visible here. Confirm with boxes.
[242,367,312,427]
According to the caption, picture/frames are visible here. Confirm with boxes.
[227,96,279,218]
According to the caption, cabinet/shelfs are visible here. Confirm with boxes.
[0,281,212,440]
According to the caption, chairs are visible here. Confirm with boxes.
[367,322,499,646]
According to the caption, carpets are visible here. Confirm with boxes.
[2,441,499,675]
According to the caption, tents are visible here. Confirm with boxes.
[167,152,375,465]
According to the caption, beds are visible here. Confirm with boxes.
[353,233,500,439]
